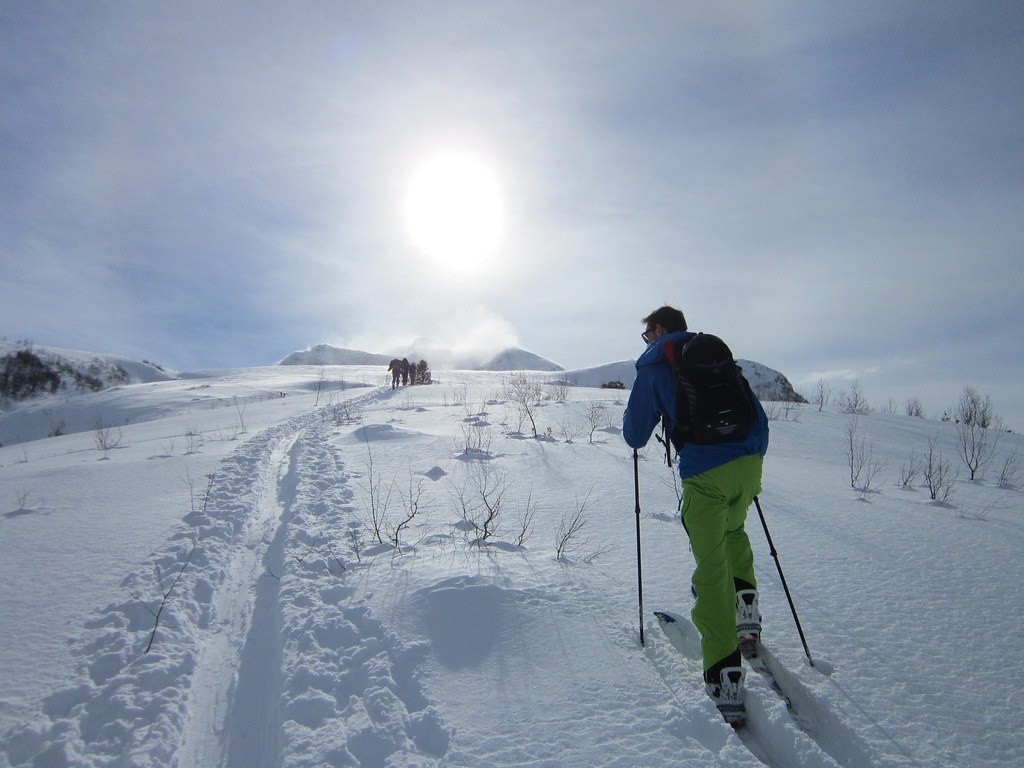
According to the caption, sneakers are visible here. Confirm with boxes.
[734,590,762,653]
[704,667,748,727]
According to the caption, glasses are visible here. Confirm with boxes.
[642,326,656,342]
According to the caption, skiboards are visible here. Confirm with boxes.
[652,602,827,766]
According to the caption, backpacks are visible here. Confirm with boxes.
[665,331,757,452]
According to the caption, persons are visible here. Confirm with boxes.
[388,358,431,390]
[623,306,770,723]
